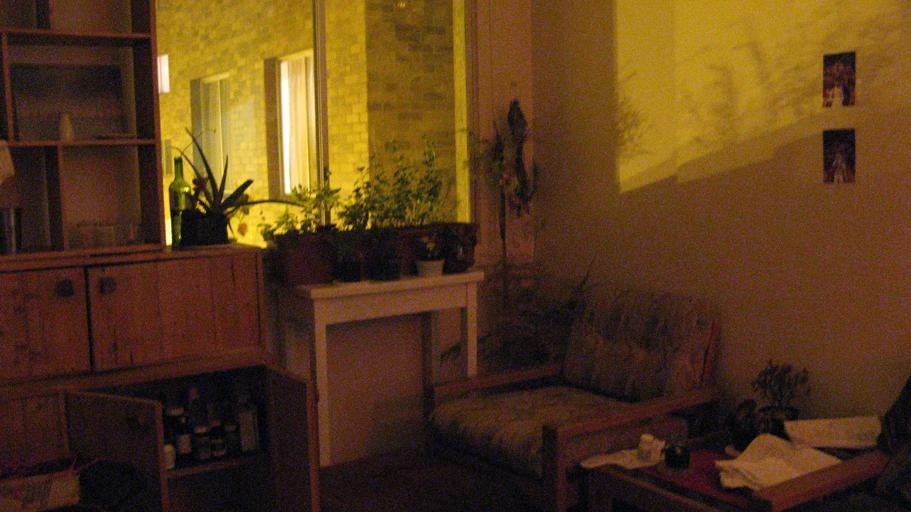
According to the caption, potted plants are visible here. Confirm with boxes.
[752,358,812,438]
[171,126,478,285]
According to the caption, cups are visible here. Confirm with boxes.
[56,109,75,141]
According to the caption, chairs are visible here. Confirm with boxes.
[428,284,721,512]
[752,368,911,512]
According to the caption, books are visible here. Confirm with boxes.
[783,414,882,452]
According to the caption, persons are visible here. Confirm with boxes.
[828,77,852,108]
[833,152,849,184]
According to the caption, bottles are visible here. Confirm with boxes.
[666,444,690,470]
[162,386,262,472]
[638,433,655,463]
[169,155,194,251]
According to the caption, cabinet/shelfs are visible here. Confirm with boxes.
[1,0,167,263]
[0,244,321,511]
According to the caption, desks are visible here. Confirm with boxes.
[578,443,751,512]
[279,271,485,466]
